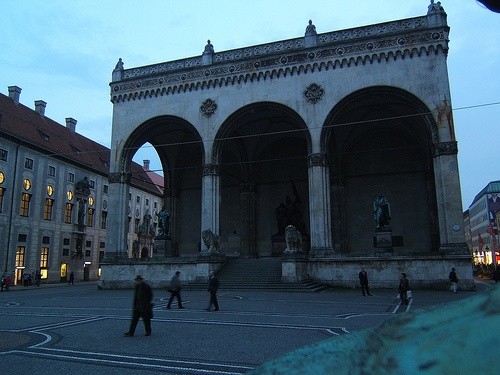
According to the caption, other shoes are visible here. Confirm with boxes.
[123,333,134,337]
[145,332,151,336]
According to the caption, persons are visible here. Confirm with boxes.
[23,271,43,287]
[427,0,445,13]
[399,273,409,305]
[0,275,11,292]
[359,266,372,296]
[205,270,219,311]
[203,40,214,54]
[449,267,457,293]
[124,275,153,337]
[155,206,169,236]
[305,20,316,36]
[477,262,495,280]
[69,272,74,285]
[376,192,390,228]
[166,271,185,309]
[115,58,124,69]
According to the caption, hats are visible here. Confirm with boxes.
[135,275,143,280]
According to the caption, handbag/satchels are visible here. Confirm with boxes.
[407,291,412,297]
